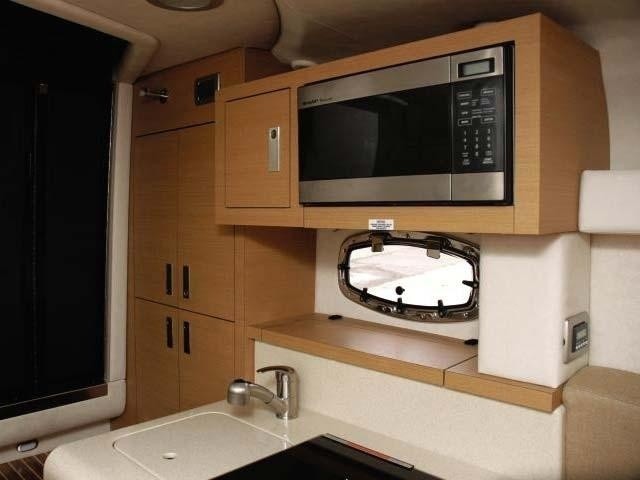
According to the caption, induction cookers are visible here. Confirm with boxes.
[204,431,452,480]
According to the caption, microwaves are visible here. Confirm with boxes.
[297,44,514,209]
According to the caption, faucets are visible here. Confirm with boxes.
[226,364,300,419]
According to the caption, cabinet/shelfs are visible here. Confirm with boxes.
[127,120,315,425]
